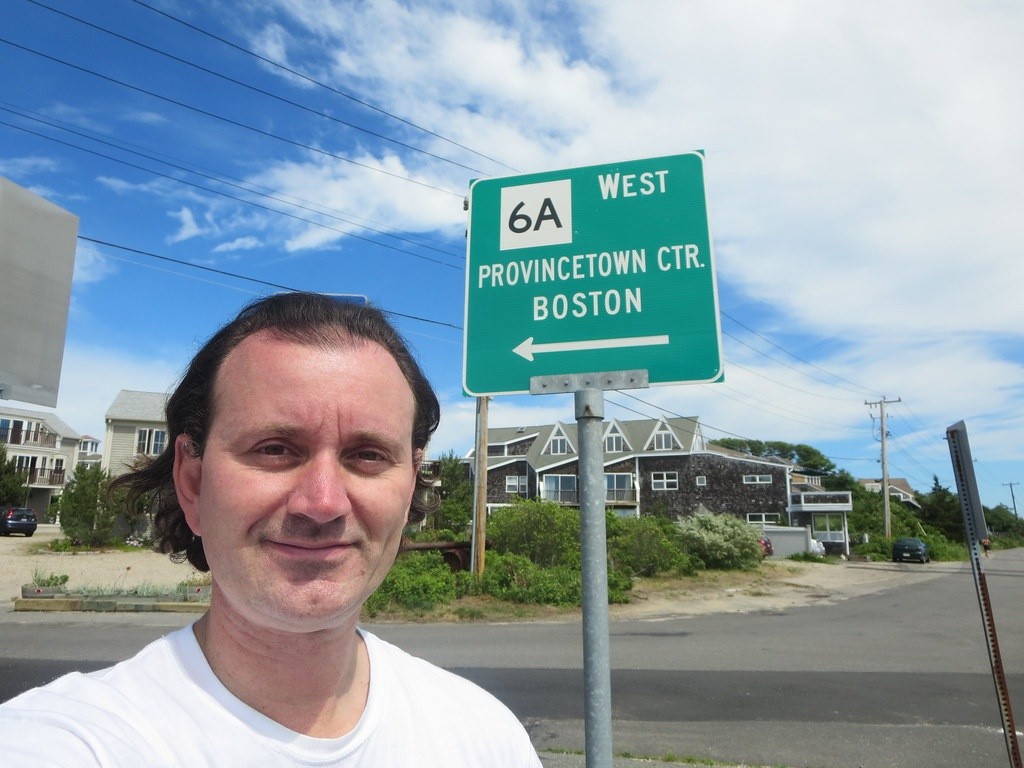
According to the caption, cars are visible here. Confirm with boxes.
[892,538,930,564]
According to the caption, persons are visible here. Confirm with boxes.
[980,534,995,559]
[0,290,546,768]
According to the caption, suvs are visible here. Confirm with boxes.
[0,507,38,537]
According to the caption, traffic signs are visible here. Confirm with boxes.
[457,148,725,398]
